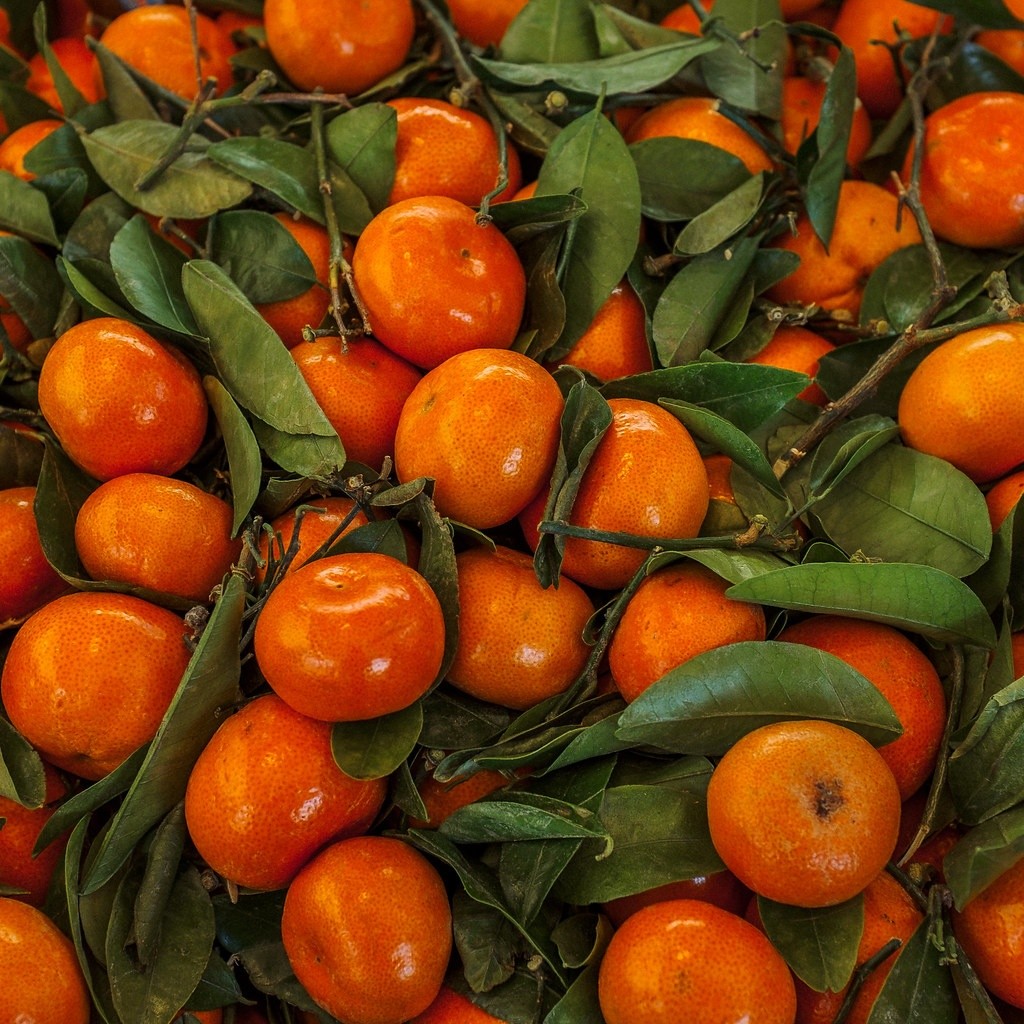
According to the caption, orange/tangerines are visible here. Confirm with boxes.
[0,0,1024,1024]
